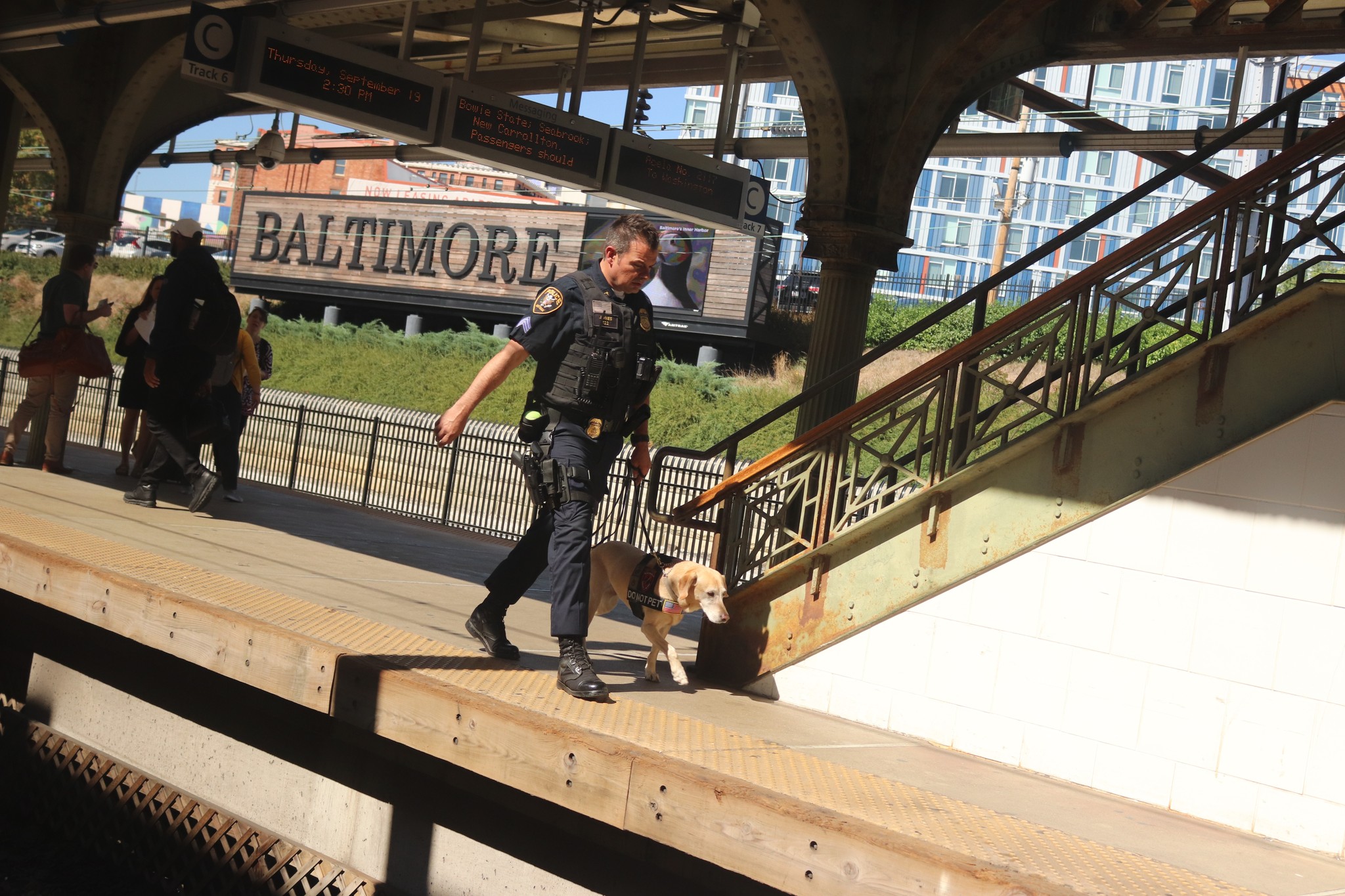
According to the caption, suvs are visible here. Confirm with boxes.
[1,227,66,251]
[774,271,821,313]
[110,235,173,259]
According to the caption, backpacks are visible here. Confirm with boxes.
[194,292,241,356]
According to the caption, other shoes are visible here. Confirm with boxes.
[188,470,221,512]
[0,452,15,466]
[125,484,159,508]
[42,460,73,474]
[131,466,144,478]
[223,489,243,502]
[115,465,129,475]
[214,471,222,483]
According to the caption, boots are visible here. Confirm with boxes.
[465,594,522,659]
[556,637,611,698]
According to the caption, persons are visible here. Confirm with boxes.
[0,245,113,475]
[115,274,167,479]
[123,218,228,512]
[161,308,273,501]
[434,214,663,699]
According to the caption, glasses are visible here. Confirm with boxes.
[253,306,269,316]
[91,261,99,269]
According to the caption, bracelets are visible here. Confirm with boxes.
[253,390,261,396]
[630,433,649,447]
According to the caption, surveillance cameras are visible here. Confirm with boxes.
[256,132,285,170]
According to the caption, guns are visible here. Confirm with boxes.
[511,450,545,505]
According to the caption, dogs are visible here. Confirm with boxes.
[581,540,731,687]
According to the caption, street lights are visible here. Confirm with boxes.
[134,171,141,194]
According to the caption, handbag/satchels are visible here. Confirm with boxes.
[19,339,61,378]
[55,328,115,378]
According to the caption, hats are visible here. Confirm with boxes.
[163,218,204,238]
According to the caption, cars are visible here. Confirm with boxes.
[15,236,65,264]
[210,249,235,266]
[888,298,941,314]
